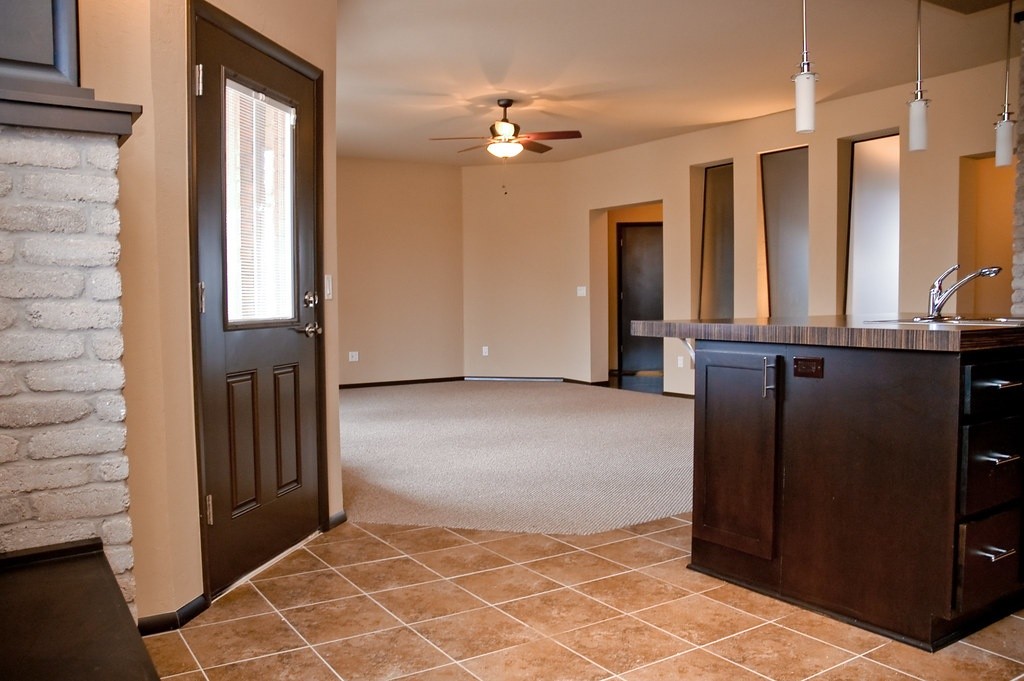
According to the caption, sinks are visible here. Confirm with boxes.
[864,318,1024,328]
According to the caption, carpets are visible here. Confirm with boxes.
[338,381,694,535]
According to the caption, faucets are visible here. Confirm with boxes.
[928,263,1002,316]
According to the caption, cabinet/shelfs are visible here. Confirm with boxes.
[687,339,784,598]
[785,342,1024,655]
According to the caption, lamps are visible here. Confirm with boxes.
[791,0,820,134]
[905,0,933,152]
[487,98,521,196]
[993,0,1018,168]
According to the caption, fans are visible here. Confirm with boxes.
[427,98,582,153]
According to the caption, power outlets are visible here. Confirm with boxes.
[483,346,488,355]
[349,352,358,361]
[678,356,683,367]
